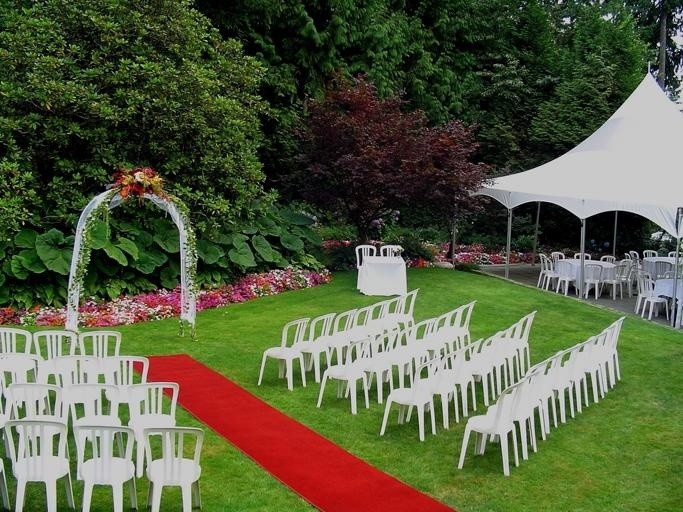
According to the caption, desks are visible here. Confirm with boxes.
[357,255,410,297]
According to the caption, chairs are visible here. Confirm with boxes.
[406,310,539,429]
[540,244,681,336]
[325,289,420,365]
[379,244,403,260]
[367,300,478,391]
[353,245,377,267]
[380,355,444,441]
[0,325,207,511]
[301,313,336,383]
[316,337,370,415]
[345,331,400,404]
[480,316,624,461]
[458,378,528,477]
[258,318,310,391]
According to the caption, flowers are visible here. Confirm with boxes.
[109,166,168,206]
